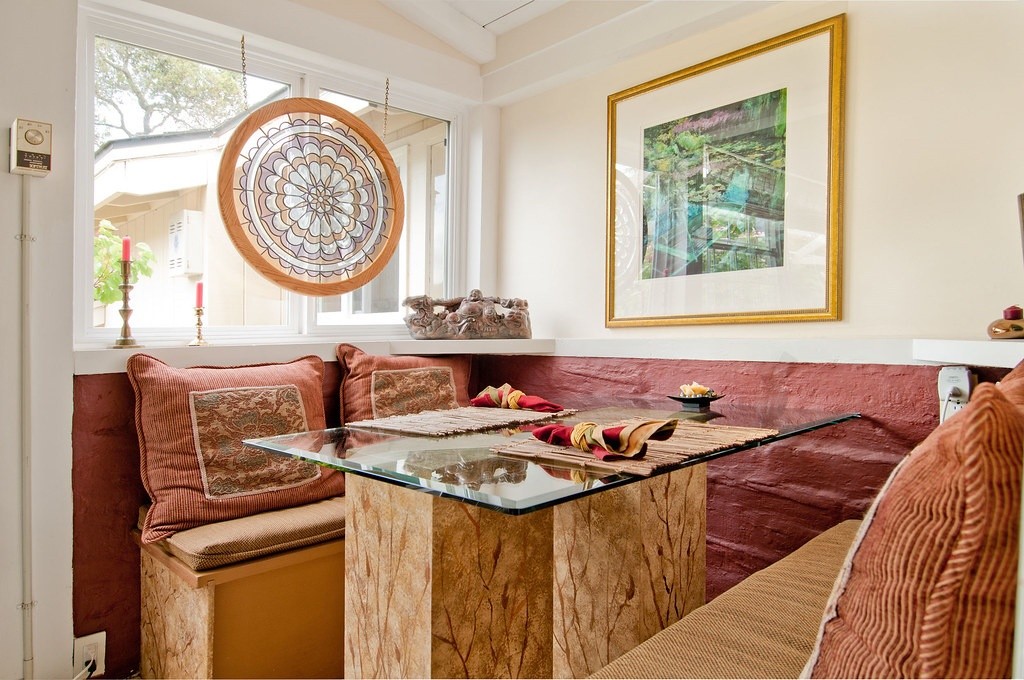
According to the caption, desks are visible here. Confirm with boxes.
[240,395,861,680]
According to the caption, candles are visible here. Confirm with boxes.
[121,234,130,260]
[195,281,204,307]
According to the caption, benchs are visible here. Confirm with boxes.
[128,502,346,680]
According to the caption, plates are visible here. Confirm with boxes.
[668,410,726,423]
[668,394,727,403]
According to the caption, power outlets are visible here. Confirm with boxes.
[73,631,106,677]
[939,372,977,428]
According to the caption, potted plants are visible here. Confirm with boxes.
[94,218,157,327]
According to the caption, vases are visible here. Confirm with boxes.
[668,392,726,413]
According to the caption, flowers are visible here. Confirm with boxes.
[679,377,711,396]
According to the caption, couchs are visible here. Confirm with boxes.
[584,520,861,680]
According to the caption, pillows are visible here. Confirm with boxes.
[801,360,1024,680]
[123,351,346,546]
[337,341,471,459]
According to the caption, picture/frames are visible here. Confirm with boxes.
[604,16,847,328]
